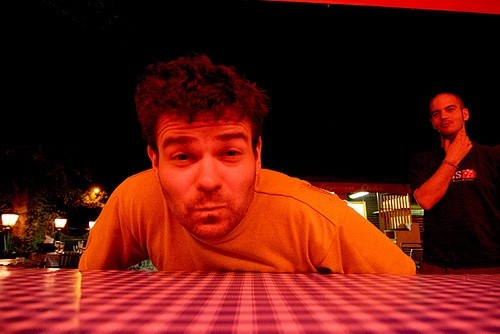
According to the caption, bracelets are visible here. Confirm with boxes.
[445,159,459,168]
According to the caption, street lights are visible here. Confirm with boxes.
[1,213,19,254]
[54,217,67,241]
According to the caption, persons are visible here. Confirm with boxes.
[408,91,500,272]
[71,55,416,276]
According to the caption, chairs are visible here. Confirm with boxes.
[55,252,83,269]
[402,243,425,269]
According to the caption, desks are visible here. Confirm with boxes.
[32,252,83,268]
[2,265,500,334]
